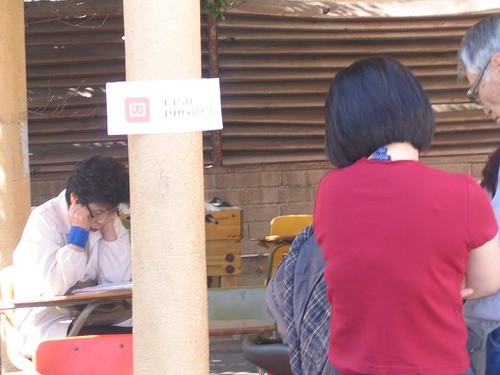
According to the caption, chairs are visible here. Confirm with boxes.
[36,331,133,375]
[262,214,312,287]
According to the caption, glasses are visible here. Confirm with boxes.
[467,58,493,105]
[85,204,120,220]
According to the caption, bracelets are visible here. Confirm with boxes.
[67,226,90,249]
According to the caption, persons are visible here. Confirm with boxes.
[10,156,134,360]
[313,14,500,375]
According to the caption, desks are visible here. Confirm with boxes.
[0,288,132,336]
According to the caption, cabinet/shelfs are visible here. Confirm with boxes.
[205,207,244,277]
